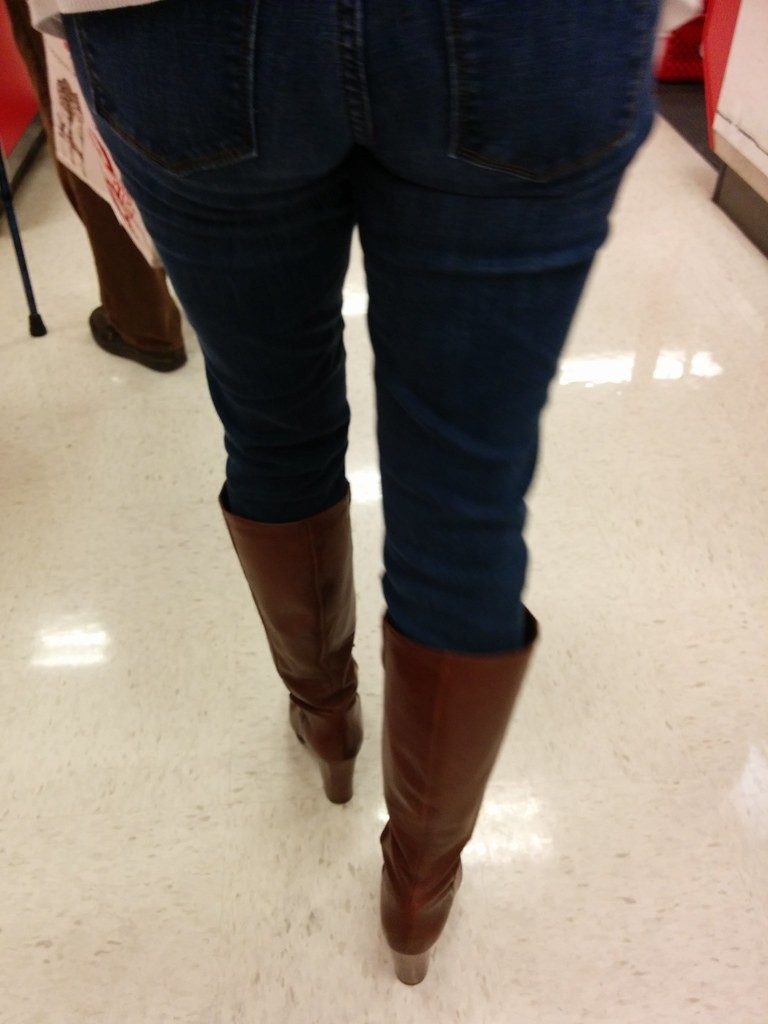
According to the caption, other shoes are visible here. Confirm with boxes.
[90,302,186,372]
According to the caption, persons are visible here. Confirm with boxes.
[5,0,189,372]
[31,1,670,983]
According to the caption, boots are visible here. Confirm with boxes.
[220,477,362,805]
[380,600,541,984]
